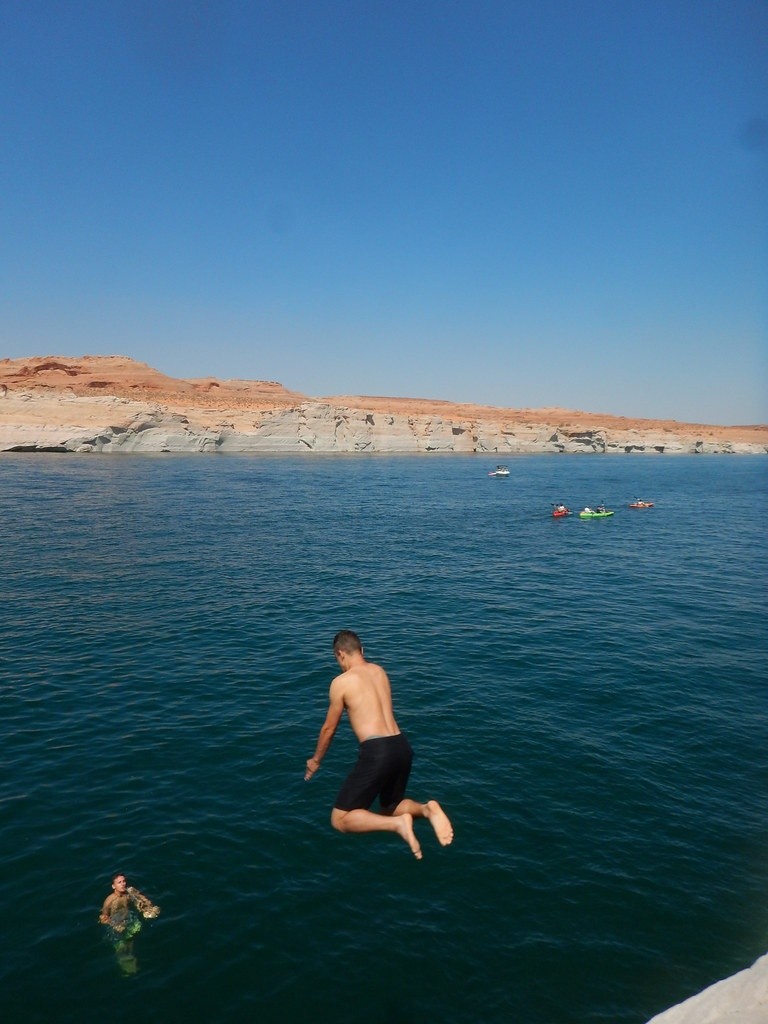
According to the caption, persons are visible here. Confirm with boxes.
[102,873,152,911]
[597,506,605,513]
[304,630,455,859]
[638,499,644,505]
[585,506,595,514]
[556,503,565,512]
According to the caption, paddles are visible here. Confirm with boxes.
[601,499,607,517]
[634,495,647,508]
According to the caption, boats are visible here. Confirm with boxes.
[580,511,615,519]
[629,502,654,508]
[553,507,572,517]
[488,464,511,477]
